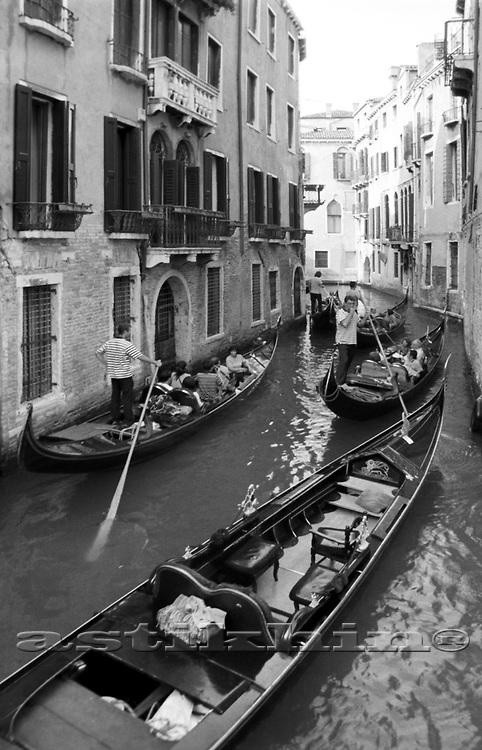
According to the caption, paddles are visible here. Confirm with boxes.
[107,362,158,520]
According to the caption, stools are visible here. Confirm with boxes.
[223,536,284,593]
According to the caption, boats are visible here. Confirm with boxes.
[319,305,447,420]
[18,311,283,474]
[0,363,450,747]
[358,285,410,342]
[308,284,344,333]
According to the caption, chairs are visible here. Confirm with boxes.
[289,563,340,611]
[309,511,370,564]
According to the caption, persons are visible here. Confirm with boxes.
[346,281,363,314]
[310,271,325,314]
[139,346,252,414]
[368,337,428,391]
[95,322,163,425]
[335,296,371,392]
[371,307,398,330]
